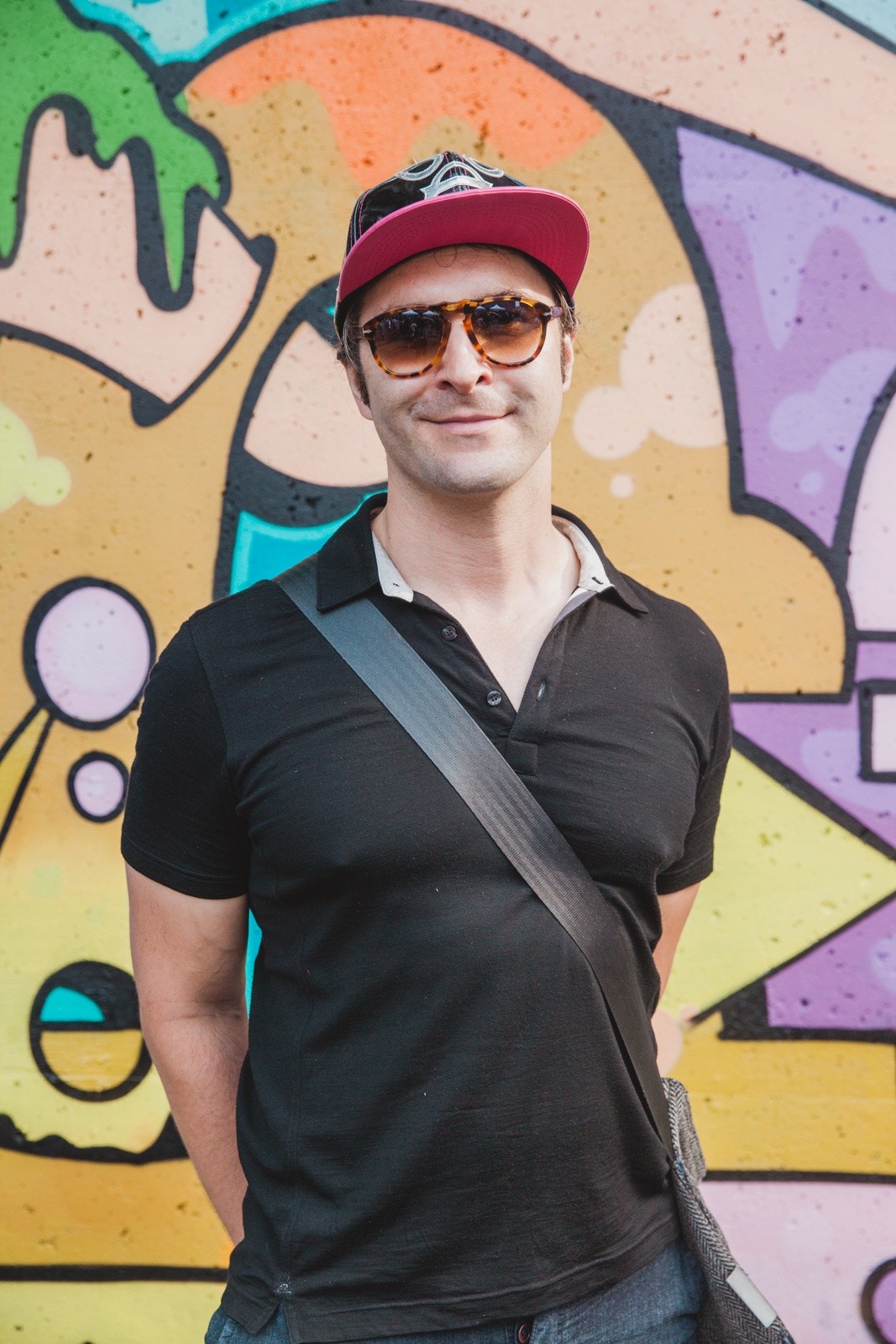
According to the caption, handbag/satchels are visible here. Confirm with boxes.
[653,1076,794,1344]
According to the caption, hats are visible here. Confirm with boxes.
[332,150,590,344]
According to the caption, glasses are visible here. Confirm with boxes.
[355,292,563,378]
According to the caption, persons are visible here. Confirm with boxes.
[129,153,733,1344]
